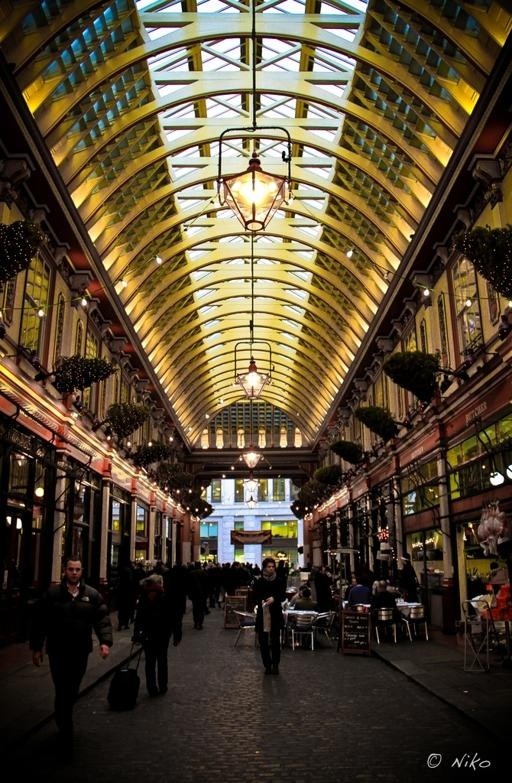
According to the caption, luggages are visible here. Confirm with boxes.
[104,637,144,712]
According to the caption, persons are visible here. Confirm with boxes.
[31,553,114,761]
[487,561,509,594]
[131,573,183,698]
[245,558,286,676]
[118,555,419,631]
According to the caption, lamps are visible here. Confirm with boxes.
[213,1,294,237]
[233,230,275,402]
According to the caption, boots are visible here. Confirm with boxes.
[263,659,279,675]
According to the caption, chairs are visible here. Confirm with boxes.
[231,600,427,651]
